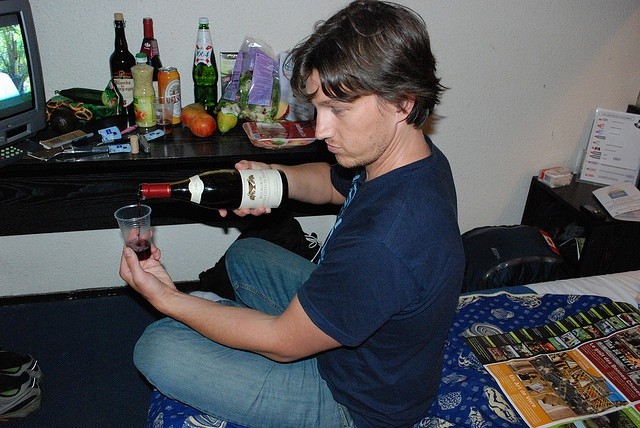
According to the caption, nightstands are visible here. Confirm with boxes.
[521,172,639,269]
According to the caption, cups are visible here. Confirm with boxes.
[114,205,152,260]
[152,97,174,126]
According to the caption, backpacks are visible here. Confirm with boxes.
[464,225,563,289]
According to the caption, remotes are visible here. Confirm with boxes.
[0,147,27,166]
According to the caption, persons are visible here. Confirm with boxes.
[118,1,466,427]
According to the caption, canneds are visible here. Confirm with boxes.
[158,67,181,124]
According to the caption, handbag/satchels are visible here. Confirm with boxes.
[199,208,322,301]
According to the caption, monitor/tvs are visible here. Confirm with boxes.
[0,17,47,143]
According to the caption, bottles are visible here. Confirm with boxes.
[137,17,165,121]
[131,53,154,127]
[139,169,288,209]
[109,13,136,120]
[192,17,218,115]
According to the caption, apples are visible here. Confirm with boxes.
[181,104,205,127]
[190,113,216,137]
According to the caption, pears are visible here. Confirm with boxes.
[218,111,238,132]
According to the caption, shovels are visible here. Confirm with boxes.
[27,145,109,162]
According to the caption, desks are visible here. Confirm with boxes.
[0,103,365,240]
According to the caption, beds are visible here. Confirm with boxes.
[145,259,640,427]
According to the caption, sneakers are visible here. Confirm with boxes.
[0,350,45,385]
[0,371,41,422]
